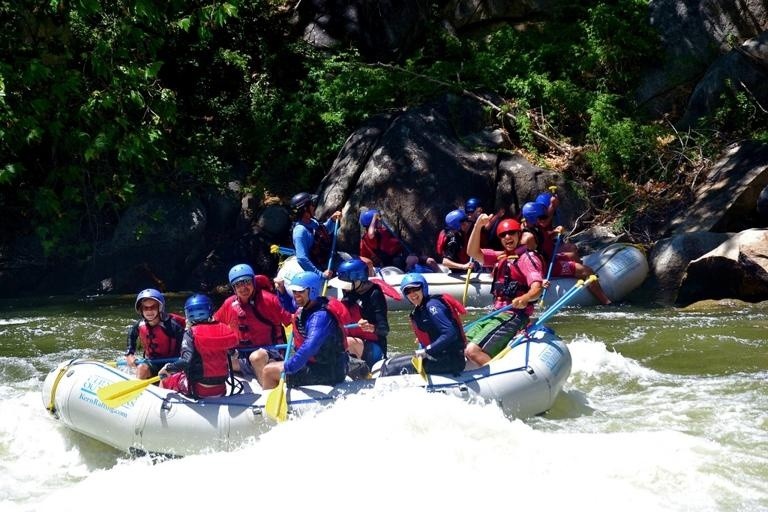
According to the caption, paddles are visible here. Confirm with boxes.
[321,217,339,296]
[265,328,293,423]
[96,369,176,408]
[462,257,473,306]
[484,275,598,364]
[411,342,428,384]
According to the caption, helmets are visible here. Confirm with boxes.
[464,197,481,213]
[290,271,322,308]
[290,192,319,217]
[400,273,429,303]
[336,257,369,285]
[445,209,467,232]
[521,202,550,226]
[183,292,214,325]
[134,288,168,321]
[495,218,522,238]
[228,264,257,297]
[535,192,552,209]
[359,209,384,228]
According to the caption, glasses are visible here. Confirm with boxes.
[142,303,160,312]
[293,290,306,294]
[499,230,518,238]
[403,285,422,295]
[234,278,251,288]
[306,202,315,207]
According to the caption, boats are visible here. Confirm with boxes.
[41,322,572,456]
[274,243,649,307]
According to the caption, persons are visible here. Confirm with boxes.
[127,193,626,399]
